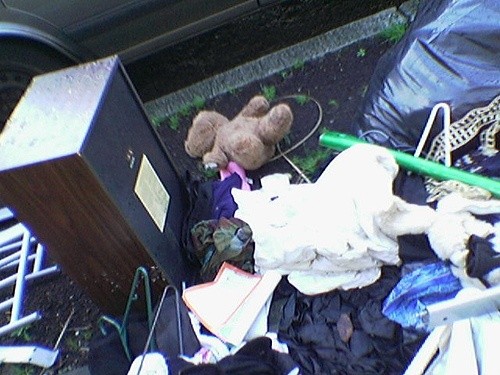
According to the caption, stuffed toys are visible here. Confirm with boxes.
[183,94,294,170]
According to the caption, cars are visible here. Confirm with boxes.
[0,0,295,132]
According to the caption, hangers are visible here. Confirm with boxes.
[96,266,156,365]
[136,284,185,375]
[407,102,451,177]
